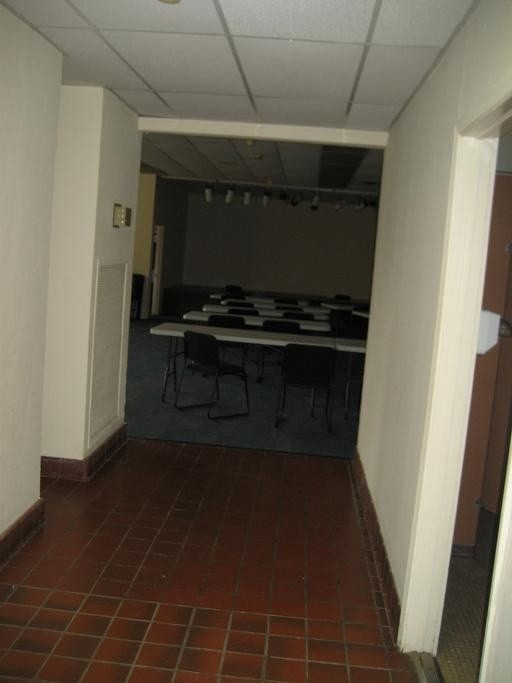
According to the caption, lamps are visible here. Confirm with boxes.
[204,179,370,211]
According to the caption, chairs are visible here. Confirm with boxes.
[174,284,369,433]
[130,273,144,321]
